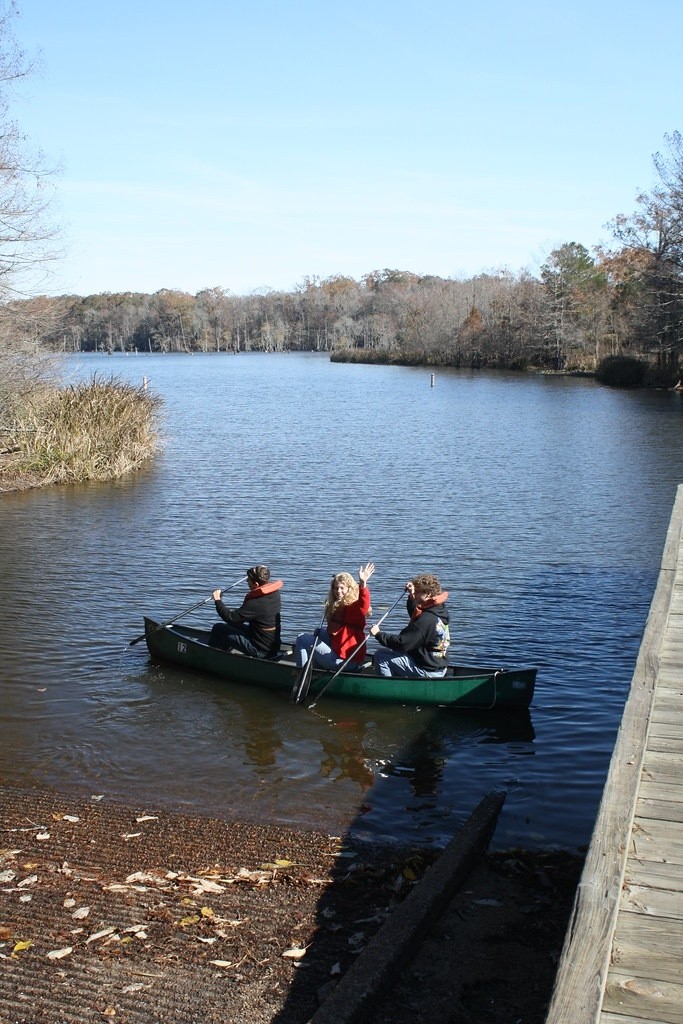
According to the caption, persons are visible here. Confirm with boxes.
[208,565,284,659]
[294,563,375,671]
[369,574,450,677]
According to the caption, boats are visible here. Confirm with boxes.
[143,615,538,711]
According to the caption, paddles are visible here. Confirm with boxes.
[292,603,327,704]
[130,576,248,645]
[310,586,409,708]
